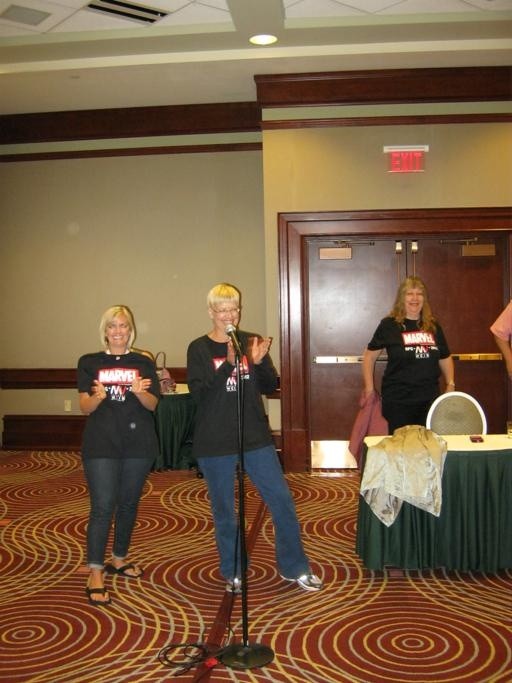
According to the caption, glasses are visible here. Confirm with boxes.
[210,306,240,315]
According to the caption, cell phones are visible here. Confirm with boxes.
[470,436,483,442]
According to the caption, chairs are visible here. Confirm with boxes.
[424,392,487,435]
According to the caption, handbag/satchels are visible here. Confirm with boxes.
[152,352,176,393]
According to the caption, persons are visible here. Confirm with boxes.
[75,302,163,606]
[186,282,324,594]
[359,275,455,435]
[488,298,511,379]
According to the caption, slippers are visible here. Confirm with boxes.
[109,563,144,578]
[86,583,111,605]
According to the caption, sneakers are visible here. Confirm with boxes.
[224,575,243,593]
[280,568,323,590]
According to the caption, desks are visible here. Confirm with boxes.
[151,383,193,473]
[355,432,512,577]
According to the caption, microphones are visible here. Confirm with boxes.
[225,324,241,351]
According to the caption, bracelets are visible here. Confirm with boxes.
[446,381,455,386]
